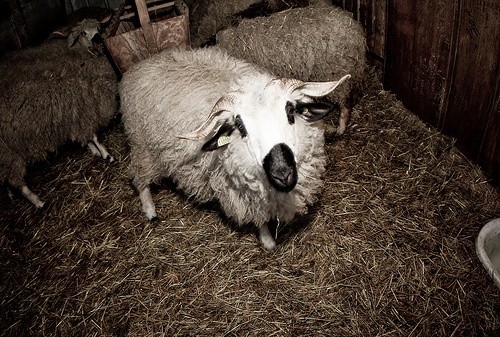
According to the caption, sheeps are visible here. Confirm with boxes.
[187,0,373,138]
[117,43,352,251]
[0,7,125,211]
